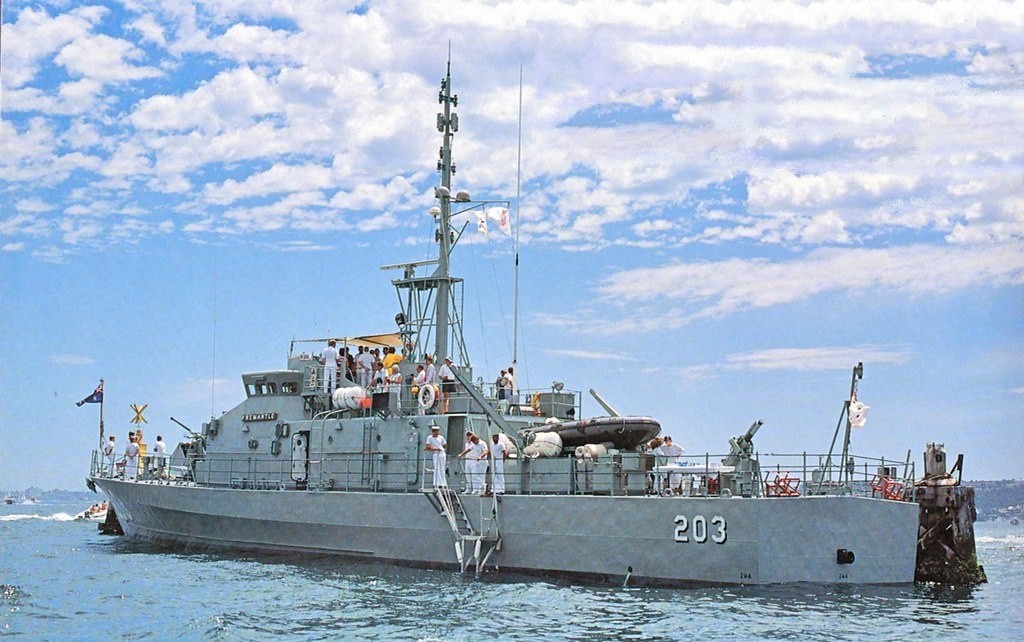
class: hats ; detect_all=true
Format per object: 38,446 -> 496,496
467,431 -> 473,434
444,356 -> 453,363
492,433 -> 499,435
431,426 -> 440,430
471,436 -> 479,440
664,436 -> 671,440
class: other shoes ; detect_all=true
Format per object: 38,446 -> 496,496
490,491 -> 503,494
478,491 -> 485,496
472,491 -> 478,495
462,490 -> 471,495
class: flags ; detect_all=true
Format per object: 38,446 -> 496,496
475,210 -> 489,233
486,207 -> 512,236
76,382 -> 103,407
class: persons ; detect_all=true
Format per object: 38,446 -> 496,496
125,432 -> 140,479
437,355 -> 456,413
388,364 -> 402,416
496,367 -> 514,401
89,500 -> 108,515
489,434 -> 508,495
321,341 -> 338,394
412,357 -> 437,414
367,362 -> 390,415
153,435 -> 167,474
648,436 -> 685,496
335,344 -> 409,388
103,435 -> 119,478
457,431 -> 490,495
425,426 -> 447,488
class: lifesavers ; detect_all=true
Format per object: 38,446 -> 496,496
344,386 -> 366,411
338,387 -> 352,409
417,384 -> 439,410
332,388 -> 343,409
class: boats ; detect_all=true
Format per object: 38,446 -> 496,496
77,35 -> 920,585
